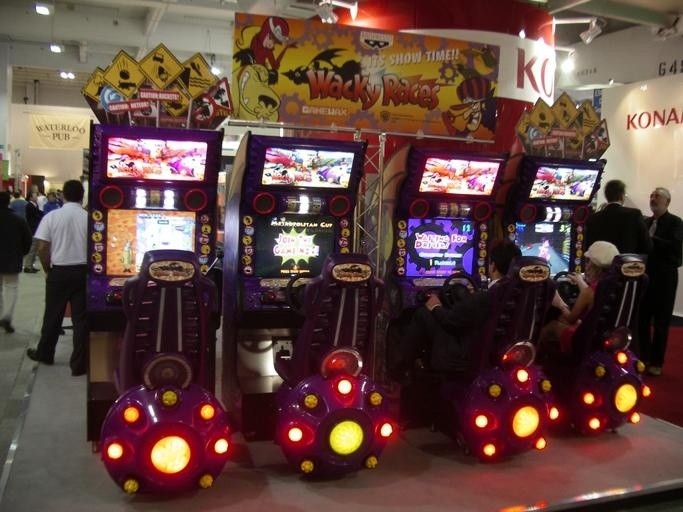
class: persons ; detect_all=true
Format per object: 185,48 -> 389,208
637,187 -> 683,375
586,180 -> 653,357
0,179 -> 88,377
386,237 -> 522,374
539,238 -> 621,357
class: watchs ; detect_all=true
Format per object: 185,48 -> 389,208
431,306 -> 439,311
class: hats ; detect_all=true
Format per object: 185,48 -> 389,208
584,240 -> 619,268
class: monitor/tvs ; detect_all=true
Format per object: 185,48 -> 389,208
405,218 -> 475,278
256,214 -> 336,277
107,208 -> 196,278
515,222 -> 572,277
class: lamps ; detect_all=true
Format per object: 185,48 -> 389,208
550,14 -> 606,45
312,0 -> 362,24
554,44 -> 578,75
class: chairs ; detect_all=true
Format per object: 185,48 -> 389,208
429,252 -> 553,397
111,242 -> 221,397
272,248 -> 391,392
557,249 -> 654,366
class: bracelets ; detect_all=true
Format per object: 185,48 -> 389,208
559,304 -> 568,310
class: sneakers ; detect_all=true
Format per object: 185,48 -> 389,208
27,348 -> 53,364
24,267 -> 39,273
0,320 -> 14,333
